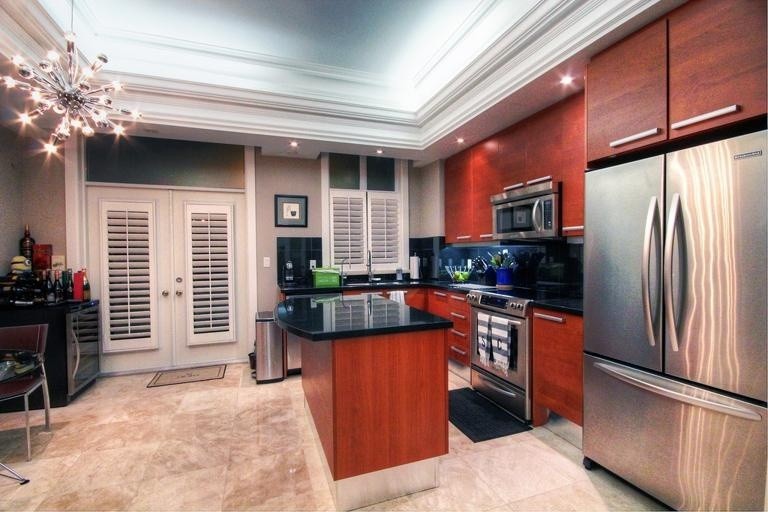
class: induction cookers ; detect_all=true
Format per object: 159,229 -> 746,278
465,288 -> 579,318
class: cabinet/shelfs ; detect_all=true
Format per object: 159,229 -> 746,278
0,300 -> 101,413
343,291 -> 384,299
496,104 -> 559,193
558,87 -> 586,237
427,289 -> 472,368
384,289 -> 426,311
443,136 -> 497,244
531,312 -> 584,426
586,1 -> 767,170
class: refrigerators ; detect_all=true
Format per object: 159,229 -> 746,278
580,124 -> 767,510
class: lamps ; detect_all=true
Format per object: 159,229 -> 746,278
0,0 -> 143,160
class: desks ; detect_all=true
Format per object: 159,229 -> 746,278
0,347 -> 49,487
270,292 -> 455,511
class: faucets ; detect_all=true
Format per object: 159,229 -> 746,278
366,250 -> 373,277
342,292 -> 349,310
366,294 -> 373,316
340,258 -> 351,283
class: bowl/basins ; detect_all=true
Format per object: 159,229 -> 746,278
444,265 -> 475,284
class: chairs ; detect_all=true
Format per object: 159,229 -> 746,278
0,323 -> 51,462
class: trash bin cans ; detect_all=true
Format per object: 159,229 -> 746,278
255,311 -> 284,384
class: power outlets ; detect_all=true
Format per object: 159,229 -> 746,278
310,260 -> 316,270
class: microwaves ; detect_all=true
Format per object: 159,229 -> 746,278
490,182 -> 560,240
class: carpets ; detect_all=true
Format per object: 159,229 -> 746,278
447,386 -> 535,444
147,362 -> 228,388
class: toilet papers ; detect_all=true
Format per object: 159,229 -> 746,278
409,257 -> 420,279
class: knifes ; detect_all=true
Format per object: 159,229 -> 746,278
471,255 -> 488,272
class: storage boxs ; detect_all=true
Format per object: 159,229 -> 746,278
313,266 -> 341,289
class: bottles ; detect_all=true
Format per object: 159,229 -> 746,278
38,267 -> 91,304
19,223 -> 36,260
396,268 -> 403,280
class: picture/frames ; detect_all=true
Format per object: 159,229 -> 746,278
274,195 -> 309,228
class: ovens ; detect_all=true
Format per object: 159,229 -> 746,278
469,307 -> 533,428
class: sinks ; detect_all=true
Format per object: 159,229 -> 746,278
346,282 -> 372,288
376,281 -> 404,288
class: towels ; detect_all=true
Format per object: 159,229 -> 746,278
490,315 -> 511,379
477,311 -> 490,369
390,292 -> 405,305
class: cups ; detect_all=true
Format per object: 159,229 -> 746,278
31,243 -> 52,272
494,268 -> 514,291
